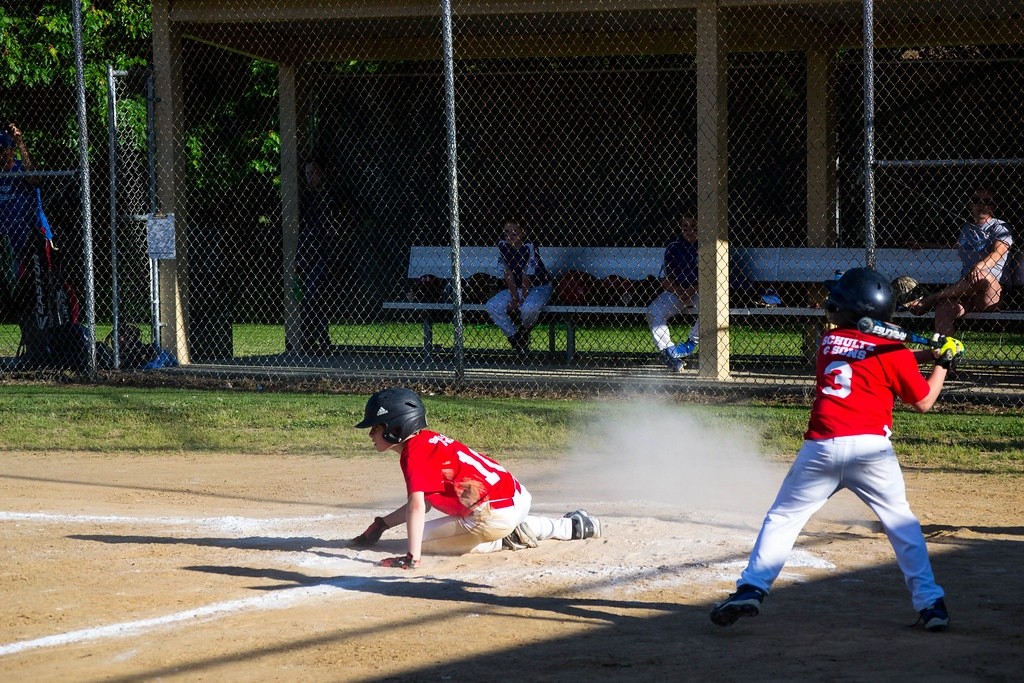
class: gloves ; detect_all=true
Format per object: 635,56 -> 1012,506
350,516 -> 388,549
930,332 -> 965,370
377,551 -> 416,570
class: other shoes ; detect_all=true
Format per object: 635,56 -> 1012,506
906,299 -> 932,316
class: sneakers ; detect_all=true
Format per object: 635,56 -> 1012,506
563,509 -> 602,541
666,343 -> 694,358
710,583 -> 765,628
669,355 -> 686,373
502,523 -> 541,550
920,598 -> 950,630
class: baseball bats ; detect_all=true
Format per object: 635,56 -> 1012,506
857,317 -> 942,348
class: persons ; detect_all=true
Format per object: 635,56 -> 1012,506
486,219 -> 552,358
902,188 -> 1012,382
0,123 -> 52,256
711,266 -> 949,629
349,386 -> 601,569
296,159 -> 338,355
646,214 -> 699,371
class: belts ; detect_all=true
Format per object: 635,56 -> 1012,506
489,480 -> 521,509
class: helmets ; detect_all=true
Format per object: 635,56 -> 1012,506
353,386 -> 430,444
824,268 -> 894,323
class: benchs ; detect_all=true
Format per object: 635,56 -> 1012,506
384,246 -> 1024,367
